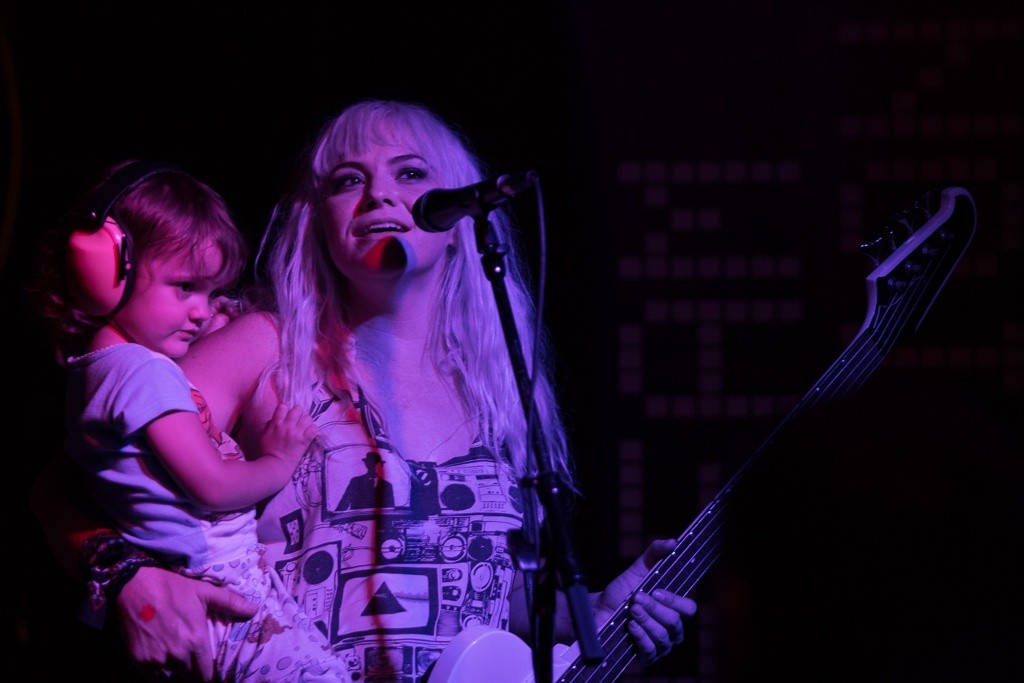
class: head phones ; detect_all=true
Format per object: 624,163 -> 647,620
55,161 -> 189,319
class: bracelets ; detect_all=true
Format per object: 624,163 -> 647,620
76,530 -> 162,613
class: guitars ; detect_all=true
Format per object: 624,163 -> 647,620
424,179 -> 980,683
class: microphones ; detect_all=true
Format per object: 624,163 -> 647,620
412,169 -> 537,234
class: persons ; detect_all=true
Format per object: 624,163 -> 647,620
85,95 -> 701,683
51,161 -> 358,683
